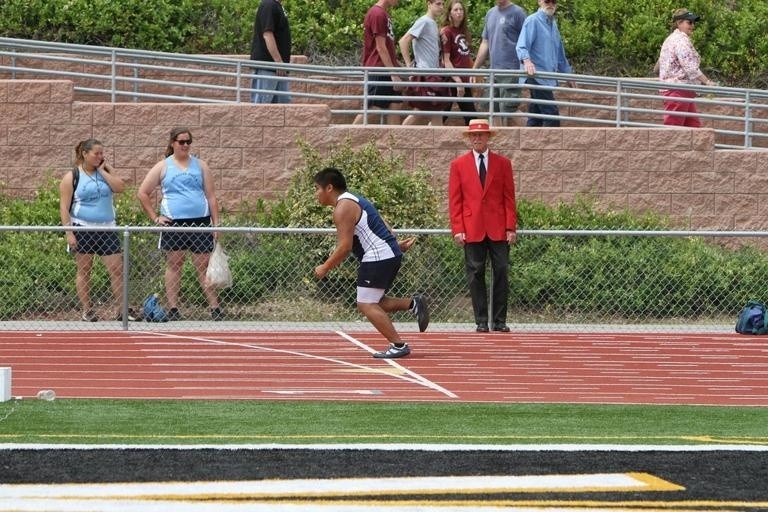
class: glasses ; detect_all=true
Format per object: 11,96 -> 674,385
175,139 -> 192,145
545,0 -> 558,4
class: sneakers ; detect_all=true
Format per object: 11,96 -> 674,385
114,308 -> 142,321
81,310 -> 104,321
210,308 -> 223,320
409,295 -> 429,332
168,308 -> 183,321
373,343 -> 410,358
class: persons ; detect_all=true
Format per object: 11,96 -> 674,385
515,0 -> 576,127
450,119 -> 516,332
399,0 -> 446,125
313,168 -> 429,358
137,128 -> 222,320
60,139 -> 135,322
653,10 -> 715,126
469,0 -> 527,126
351,0 -> 401,124
251,0 -> 291,103
441,0 -> 477,126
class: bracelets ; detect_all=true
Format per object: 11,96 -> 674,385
707,80 -> 710,85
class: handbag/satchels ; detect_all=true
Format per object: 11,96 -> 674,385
735,305 -> 768,335
408,76 -> 453,111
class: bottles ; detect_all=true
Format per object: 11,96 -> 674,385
36,390 -> 56,401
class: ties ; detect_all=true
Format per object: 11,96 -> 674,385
479,155 -> 486,185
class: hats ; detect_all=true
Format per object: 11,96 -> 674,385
462,119 -> 496,137
672,12 -> 700,22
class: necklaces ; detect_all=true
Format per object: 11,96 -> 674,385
81,163 -> 100,193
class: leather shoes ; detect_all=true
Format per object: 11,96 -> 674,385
492,322 -> 509,332
477,323 -> 489,332
